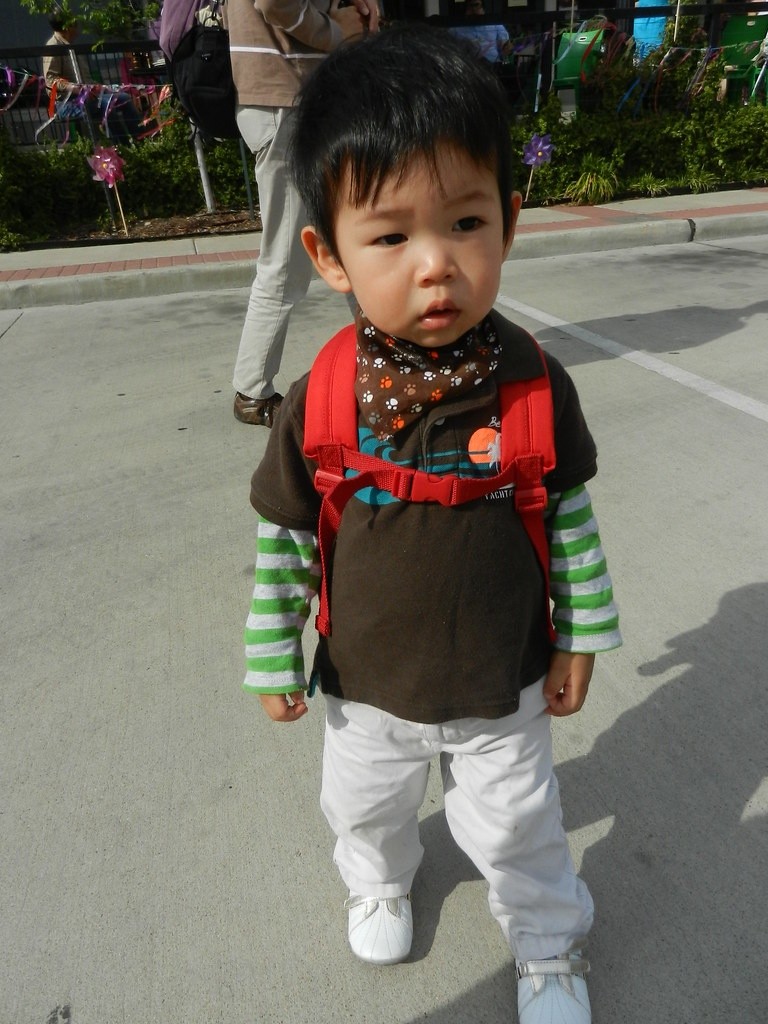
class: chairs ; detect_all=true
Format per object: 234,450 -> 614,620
718,14 -> 768,106
551,28 -> 605,121
44,86 -> 84,144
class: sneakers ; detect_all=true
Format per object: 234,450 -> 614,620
513,953 -> 592,1024
234,388 -> 283,428
347,888 -> 411,964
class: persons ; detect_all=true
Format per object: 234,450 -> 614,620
43,12 -> 93,102
225,0 -> 385,426
232,23 -> 625,1024
451,0 -> 508,69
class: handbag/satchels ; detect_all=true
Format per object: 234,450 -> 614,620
172,19 -> 241,138
